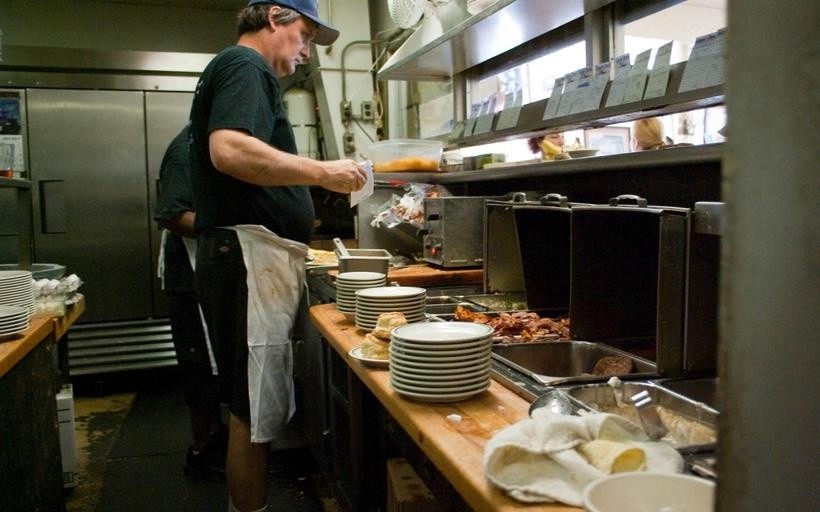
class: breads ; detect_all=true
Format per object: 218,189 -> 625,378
372,312 -> 408,340
360,333 -> 389,361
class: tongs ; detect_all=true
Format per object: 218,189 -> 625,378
631,388 -> 670,444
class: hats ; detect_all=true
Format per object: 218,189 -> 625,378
247,0 -> 340,47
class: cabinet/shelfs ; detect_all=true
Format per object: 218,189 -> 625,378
370,0 -> 729,188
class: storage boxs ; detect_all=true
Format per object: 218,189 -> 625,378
386,456 -> 444,512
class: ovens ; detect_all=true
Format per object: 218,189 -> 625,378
22,85 -> 195,378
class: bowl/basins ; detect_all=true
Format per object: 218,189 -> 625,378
582,469 -> 716,512
365,138 -> 445,174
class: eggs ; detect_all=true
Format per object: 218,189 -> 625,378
32,274 -> 78,291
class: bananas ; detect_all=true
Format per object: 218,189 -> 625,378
540,137 -> 581,160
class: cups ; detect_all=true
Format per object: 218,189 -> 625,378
585,441 -> 646,477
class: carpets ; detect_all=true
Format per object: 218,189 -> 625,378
97,458 -> 229,510
107,385 -> 231,456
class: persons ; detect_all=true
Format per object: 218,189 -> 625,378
156,122 -> 300,482
194,0 -> 369,511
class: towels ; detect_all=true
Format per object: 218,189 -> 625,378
477,404 -> 690,510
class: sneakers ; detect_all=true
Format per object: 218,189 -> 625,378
208,422 -> 229,452
181,444 -> 227,482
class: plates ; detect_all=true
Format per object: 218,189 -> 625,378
637,441 -> 685,476
0,268 -> 38,343
564,149 -> 601,160
333,270 -> 493,406
